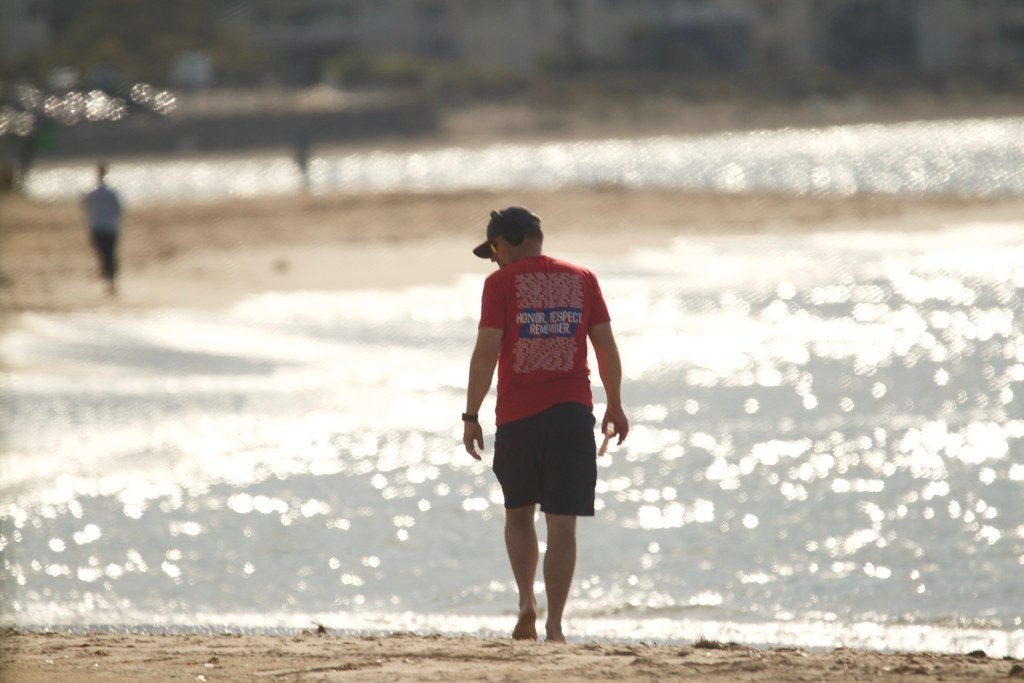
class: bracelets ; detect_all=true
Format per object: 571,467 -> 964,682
462,413 -> 479,420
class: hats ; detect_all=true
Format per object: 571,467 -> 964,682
474,206 -> 541,258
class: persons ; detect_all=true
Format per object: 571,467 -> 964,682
83,161 -> 121,296
464,207 -> 629,642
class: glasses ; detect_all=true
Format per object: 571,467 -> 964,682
489,235 -> 503,252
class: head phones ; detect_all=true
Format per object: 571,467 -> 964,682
489,209 -> 526,246
